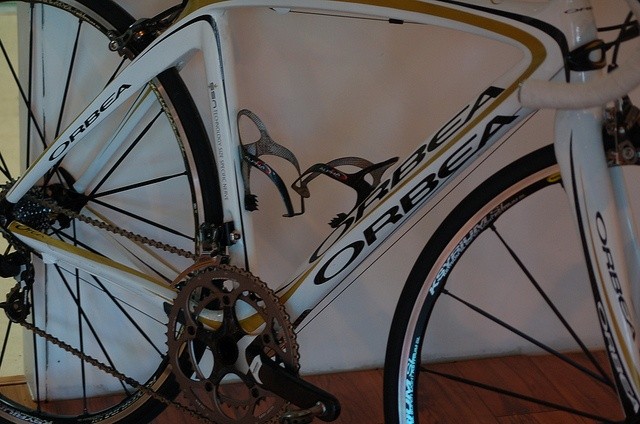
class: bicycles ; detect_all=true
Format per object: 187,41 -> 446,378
0,0 -> 640,423
